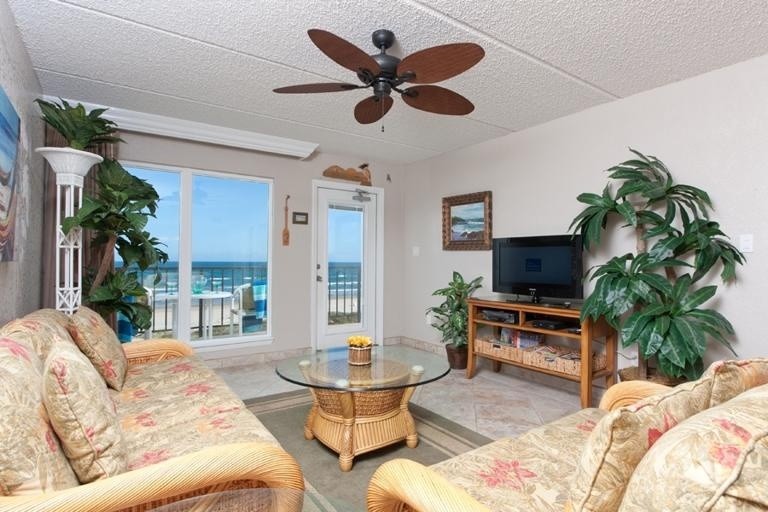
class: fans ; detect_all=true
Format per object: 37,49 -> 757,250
271,27 -> 486,133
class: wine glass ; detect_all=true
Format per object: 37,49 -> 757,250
212,281 -> 221,294
167,282 -> 177,295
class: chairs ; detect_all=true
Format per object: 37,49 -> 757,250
133,285 -> 153,345
229,282 -> 267,335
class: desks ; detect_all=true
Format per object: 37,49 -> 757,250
162,288 -> 234,339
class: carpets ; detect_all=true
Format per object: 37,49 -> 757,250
241,386 -> 498,512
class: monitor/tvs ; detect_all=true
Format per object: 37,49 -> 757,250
492,234 -> 584,307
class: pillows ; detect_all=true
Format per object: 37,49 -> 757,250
569,373 -> 712,510
701,358 -> 768,405
42,336 -> 129,487
67,302 -> 130,394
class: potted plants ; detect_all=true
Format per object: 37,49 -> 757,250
425,269 -> 482,370
567,145 -> 747,389
30,93 -> 127,187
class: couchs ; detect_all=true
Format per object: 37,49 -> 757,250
367,353 -> 767,512
0,299 -> 306,512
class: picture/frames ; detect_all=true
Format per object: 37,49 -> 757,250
441,190 -> 493,252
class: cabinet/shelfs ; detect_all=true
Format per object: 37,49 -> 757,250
464,296 -> 618,410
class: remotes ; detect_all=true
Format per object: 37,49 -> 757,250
563,300 -> 571,307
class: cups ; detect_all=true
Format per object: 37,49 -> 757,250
191,275 -> 207,294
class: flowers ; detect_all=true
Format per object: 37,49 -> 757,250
348,334 -> 377,351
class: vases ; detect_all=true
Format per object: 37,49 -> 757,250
346,346 -> 372,366
